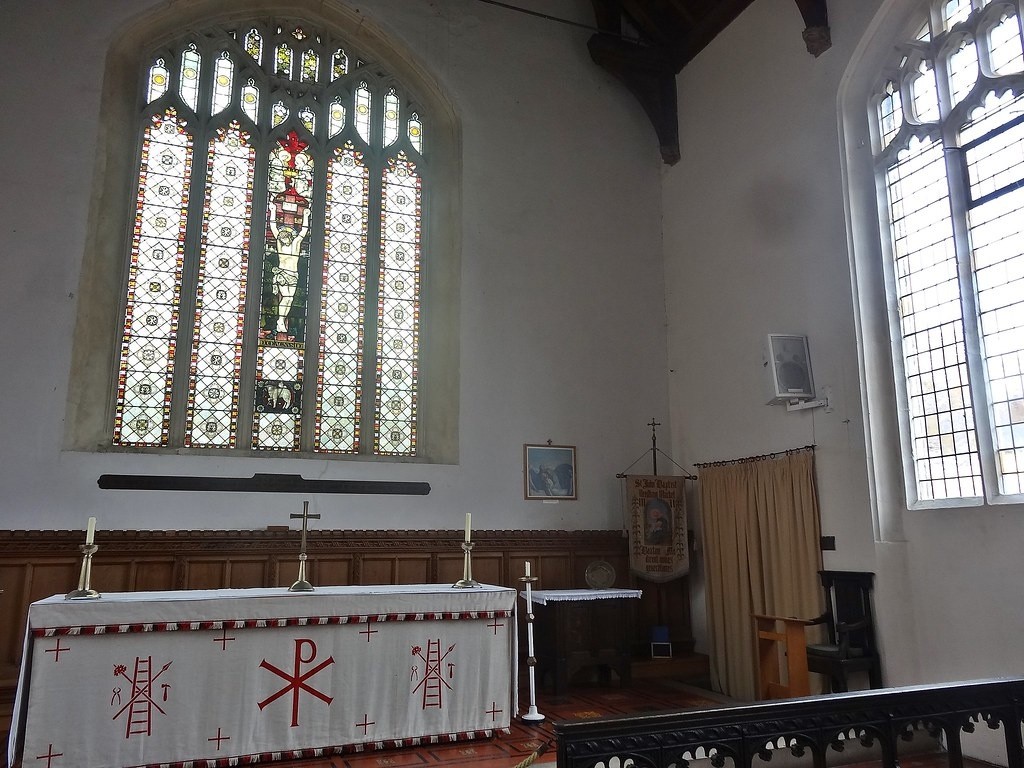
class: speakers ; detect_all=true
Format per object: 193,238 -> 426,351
761,334 -> 816,406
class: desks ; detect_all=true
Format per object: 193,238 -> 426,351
750,615 -> 814,702
5,580 -> 520,768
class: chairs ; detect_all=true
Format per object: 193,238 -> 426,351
803,568 -> 881,694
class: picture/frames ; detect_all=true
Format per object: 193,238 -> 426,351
523,443 -> 577,500
766,334 -> 816,398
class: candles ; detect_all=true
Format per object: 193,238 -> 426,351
525,561 -> 530,578
85,516 -> 96,545
465,513 -> 472,541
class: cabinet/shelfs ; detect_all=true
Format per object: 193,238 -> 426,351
518,590 -> 642,697
